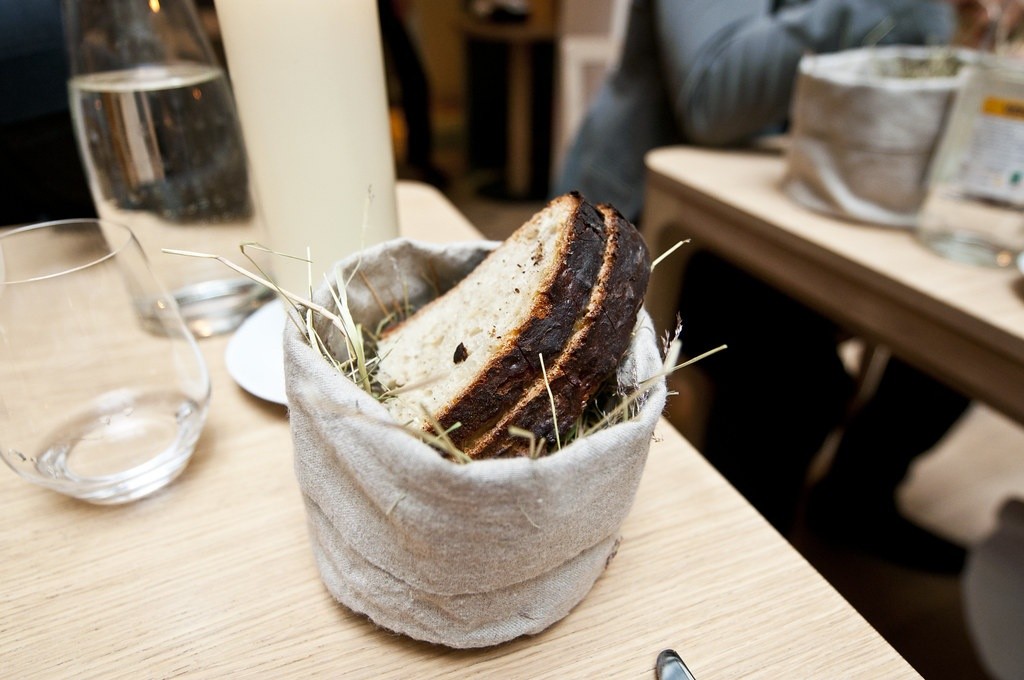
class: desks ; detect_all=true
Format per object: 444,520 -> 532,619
0,181 -> 923,680
635,135 -> 1023,452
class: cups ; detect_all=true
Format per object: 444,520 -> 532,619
0,219 -> 212,507
62,0 -> 275,341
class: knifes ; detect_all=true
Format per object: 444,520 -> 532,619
656,649 -> 695,680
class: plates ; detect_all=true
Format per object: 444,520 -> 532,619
224,298 -> 290,406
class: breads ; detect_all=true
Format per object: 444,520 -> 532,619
450,202 -> 650,460
379,190 -> 606,458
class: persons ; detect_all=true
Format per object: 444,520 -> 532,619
557,0 -> 1024,573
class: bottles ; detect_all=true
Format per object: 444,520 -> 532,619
911,0 -> 1024,271
